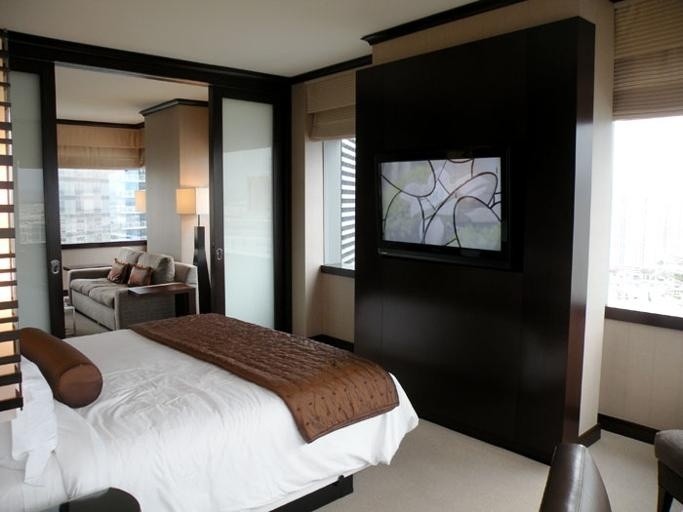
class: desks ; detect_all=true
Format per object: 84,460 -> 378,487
128,284 -> 196,317
63,264 -> 113,302
654,429 -> 682,512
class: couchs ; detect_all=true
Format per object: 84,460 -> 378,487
67,247 -> 200,330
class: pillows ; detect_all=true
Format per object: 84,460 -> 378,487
127,263 -> 152,288
107,256 -> 126,284
138,251 -> 176,282
20,324 -> 102,406
0,354 -> 57,489
45,412 -> 108,496
116,248 -> 139,268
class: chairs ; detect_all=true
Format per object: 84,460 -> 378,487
538,442 -> 611,511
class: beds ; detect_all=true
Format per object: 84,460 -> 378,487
0,311 -> 419,512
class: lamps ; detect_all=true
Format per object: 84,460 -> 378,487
176,186 -> 211,314
135,191 -> 146,213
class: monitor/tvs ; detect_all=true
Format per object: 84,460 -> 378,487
375,148 -> 511,269
